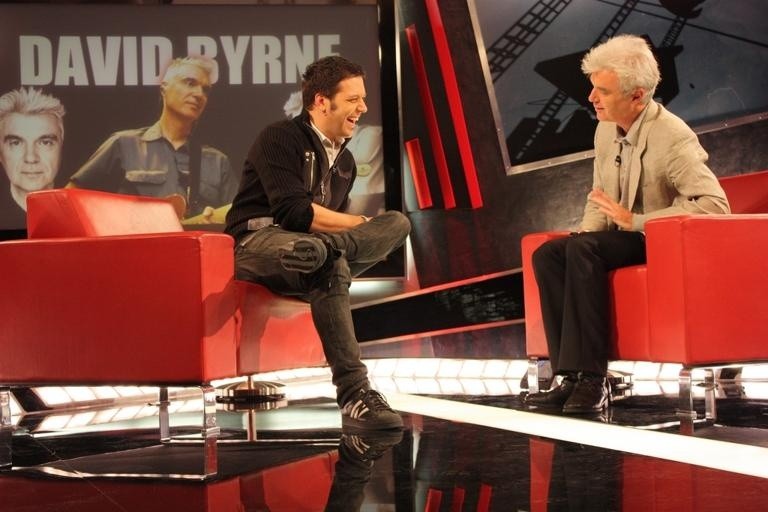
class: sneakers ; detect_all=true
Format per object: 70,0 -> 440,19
341,389 -> 403,430
340,433 -> 403,462
278,235 -> 329,273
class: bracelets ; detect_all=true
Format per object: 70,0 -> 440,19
361,214 -> 369,224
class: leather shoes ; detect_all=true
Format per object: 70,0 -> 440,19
563,374 -> 609,413
522,376 -> 573,408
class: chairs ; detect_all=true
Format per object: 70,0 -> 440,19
520,169 -> 768,435
1,188 -> 342,483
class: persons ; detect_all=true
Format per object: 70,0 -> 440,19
0,88 -> 70,242
64,54 -> 239,226
323,434 -> 409,512
523,33 -> 733,415
281,86 -> 384,214
222,54 -> 411,434
